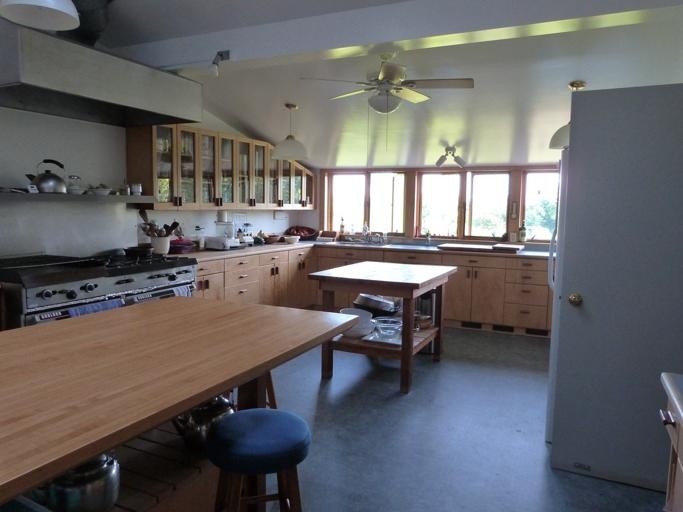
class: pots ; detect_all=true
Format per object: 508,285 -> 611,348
352,299 -> 398,316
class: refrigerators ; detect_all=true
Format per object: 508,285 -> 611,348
545,82 -> 683,492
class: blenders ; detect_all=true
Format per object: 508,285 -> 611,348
232,213 -> 254,247
206,211 -> 240,250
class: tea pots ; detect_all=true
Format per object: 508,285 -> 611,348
34,451 -> 121,511
170,388 -> 235,445
24,158 -> 67,194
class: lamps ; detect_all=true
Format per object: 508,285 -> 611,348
435,146 -> 465,168
0,0 -> 80,32
206,48 -> 231,79
368,46 -> 403,113
547,80 -> 585,150
270,101 -> 310,162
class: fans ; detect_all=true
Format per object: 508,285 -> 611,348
298,71 -> 476,105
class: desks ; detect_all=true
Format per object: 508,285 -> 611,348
307,261 -> 459,394
0,295 -> 359,511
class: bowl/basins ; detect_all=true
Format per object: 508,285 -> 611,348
284,236 -> 300,244
92,188 -> 112,196
69,187 -> 88,195
151,237 -> 170,254
340,308 -> 403,341
262,235 -> 280,245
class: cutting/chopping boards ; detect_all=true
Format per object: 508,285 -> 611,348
436,242 -> 520,254
492,243 -> 525,250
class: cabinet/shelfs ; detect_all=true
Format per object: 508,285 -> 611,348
657,373 -> 683,511
125,123 -> 316,211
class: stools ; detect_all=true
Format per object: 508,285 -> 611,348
203,408 -> 311,511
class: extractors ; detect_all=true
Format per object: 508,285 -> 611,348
0,16 -> 202,127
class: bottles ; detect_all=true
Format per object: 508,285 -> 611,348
519,220 -> 527,241
340,216 -> 344,234
363,222 -> 368,232
120,182 -> 143,196
425,229 -> 431,245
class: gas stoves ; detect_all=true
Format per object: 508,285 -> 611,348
2,246 -> 198,315
